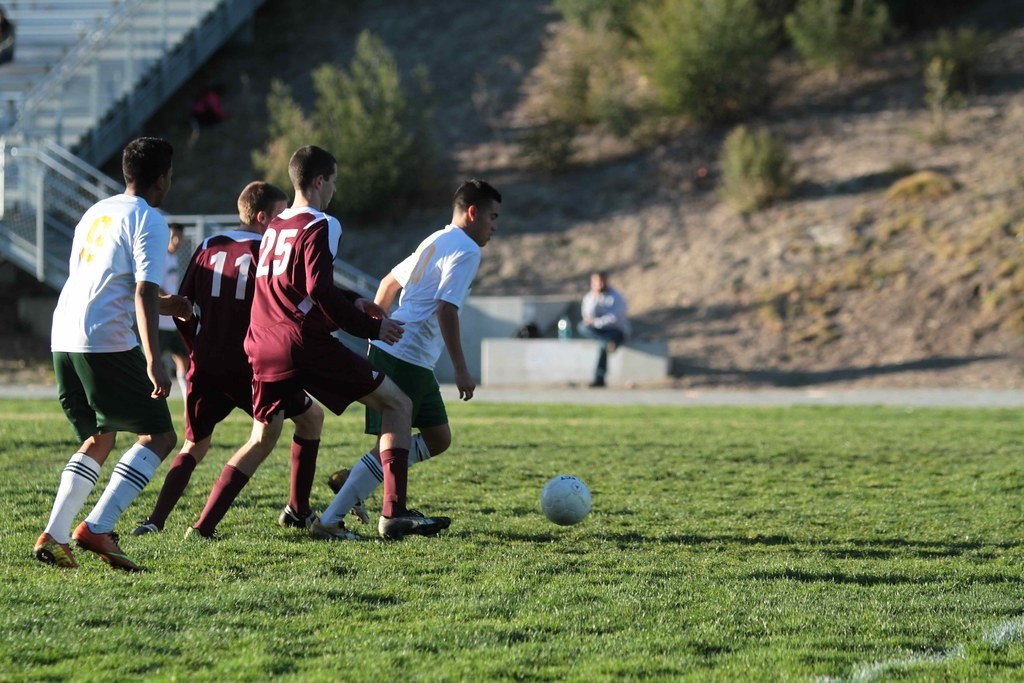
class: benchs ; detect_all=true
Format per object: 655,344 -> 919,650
480,338 -> 668,386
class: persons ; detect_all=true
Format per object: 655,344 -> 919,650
130,181 -> 326,536
34,137 -> 174,571
579,273 -> 630,388
139,223 -> 189,413
184,145 -> 452,543
310,179 -> 500,542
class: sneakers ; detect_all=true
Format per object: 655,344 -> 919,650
184,526 -> 221,541
378,509 -> 451,542
278,505 -> 321,527
134,523 -> 161,536
328,468 -> 369,524
308,516 -> 363,542
72,520 -> 138,571
32,531 -> 79,569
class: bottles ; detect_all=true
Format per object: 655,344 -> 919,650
558,314 -> 572,339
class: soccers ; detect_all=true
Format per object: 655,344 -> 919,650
540,474 -> 592,526
